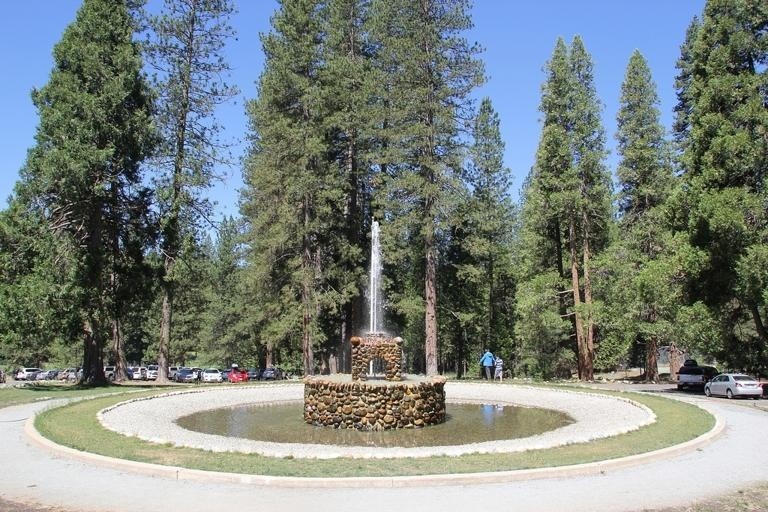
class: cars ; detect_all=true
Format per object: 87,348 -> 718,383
0,370 -> 8,383
14,364 -> 288,385
703,372 -> 764,401
684,360 -> 697,366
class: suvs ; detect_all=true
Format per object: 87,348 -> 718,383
675,366 -> 718,390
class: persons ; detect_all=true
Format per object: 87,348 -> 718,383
193,368 -> 201,386
480,349 -> 497,380
231,366 -> 246,374
493,355 -> 504,383
